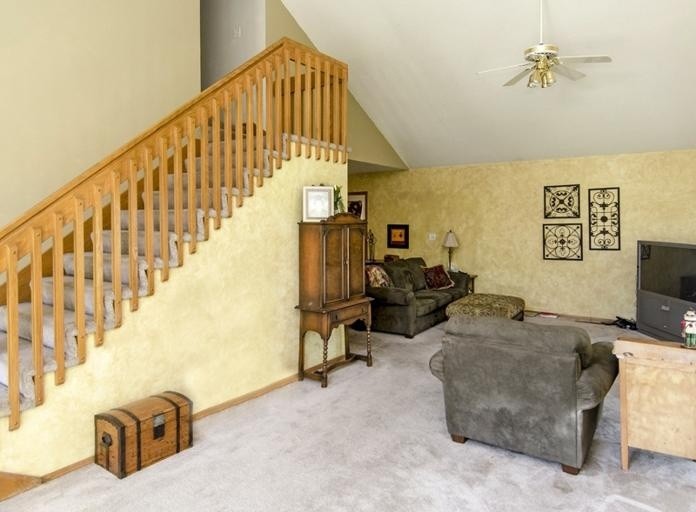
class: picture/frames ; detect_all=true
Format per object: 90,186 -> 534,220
348,191 -> 368,221
387,224 -> 410,249
303,186 -> 334,222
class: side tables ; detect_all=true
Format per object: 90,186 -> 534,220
467,274 -> 478,294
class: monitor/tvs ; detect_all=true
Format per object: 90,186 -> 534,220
635,239 -> 696,342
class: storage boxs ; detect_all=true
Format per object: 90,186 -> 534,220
94,390 -> 194,480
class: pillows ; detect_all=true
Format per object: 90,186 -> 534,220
419,264 -> 455,290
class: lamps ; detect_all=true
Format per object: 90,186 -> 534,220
442,229 -> 459,271
527,60 -> 556,89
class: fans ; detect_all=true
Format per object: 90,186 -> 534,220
477,1 -> 612,87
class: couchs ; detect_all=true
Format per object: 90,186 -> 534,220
353,256 -> 470,338
430,314 -> 620,476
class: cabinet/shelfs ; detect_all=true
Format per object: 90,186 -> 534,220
298,212 -> 375,387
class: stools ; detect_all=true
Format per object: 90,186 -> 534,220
444,293 -> 525,323
